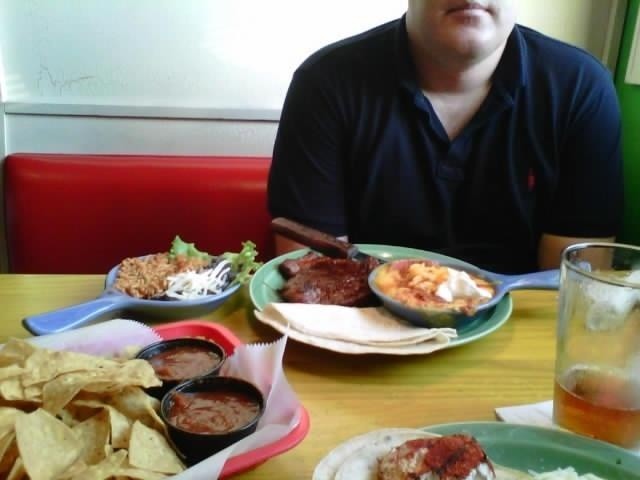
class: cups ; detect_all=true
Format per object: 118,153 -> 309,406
553,242 -> 640,451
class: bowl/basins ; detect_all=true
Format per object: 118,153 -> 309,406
135,338 -> 265,463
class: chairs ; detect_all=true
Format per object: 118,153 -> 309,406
0,152 -> 274,274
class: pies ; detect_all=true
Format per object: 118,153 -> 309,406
253,302 -> 458,355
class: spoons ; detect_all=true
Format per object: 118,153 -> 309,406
369,262 -> 590,322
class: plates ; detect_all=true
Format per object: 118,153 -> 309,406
18,252 -> 243,333
402,418 -> 640,479
250,242 -> 514,348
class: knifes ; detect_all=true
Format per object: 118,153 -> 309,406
269,217 -> 386,265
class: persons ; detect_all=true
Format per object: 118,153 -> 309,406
266,0 -> 622,271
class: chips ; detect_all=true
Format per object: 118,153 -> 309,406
0,337 -> 186,480
